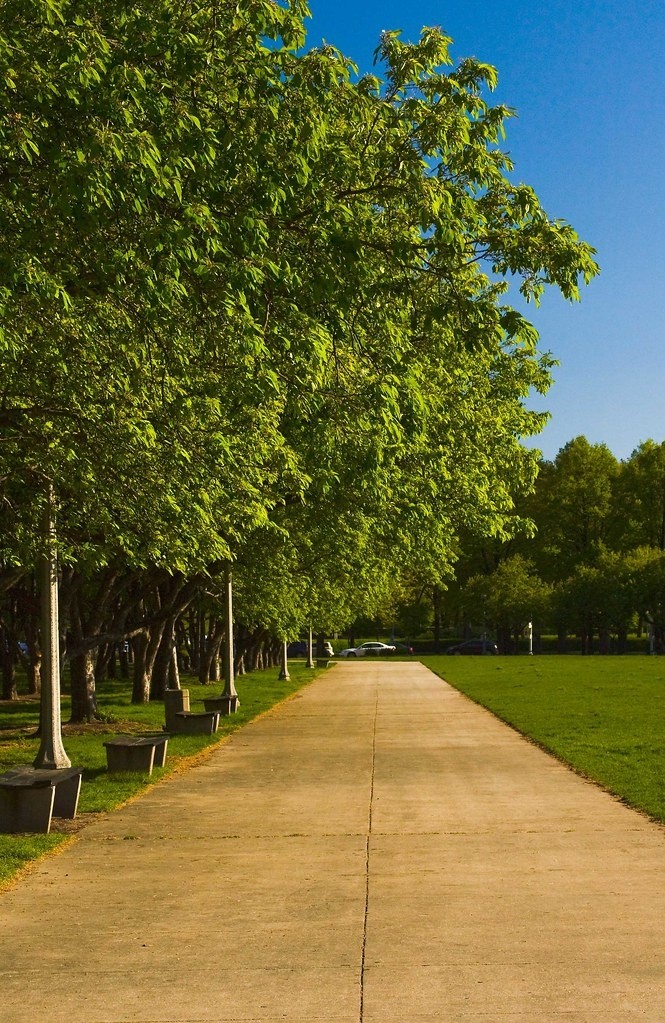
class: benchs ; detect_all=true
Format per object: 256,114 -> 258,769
178,710 -> 223,734
203,695 -> 238,715
0,768 -> 84,833
103,736 -> 169,774
316,658 -> 330,667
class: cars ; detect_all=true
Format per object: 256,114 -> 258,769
339,641 -> 396,658
440,639 -> 499,657
383,641 -> 414,656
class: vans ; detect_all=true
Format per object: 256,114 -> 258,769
287,640 -> 334,658
